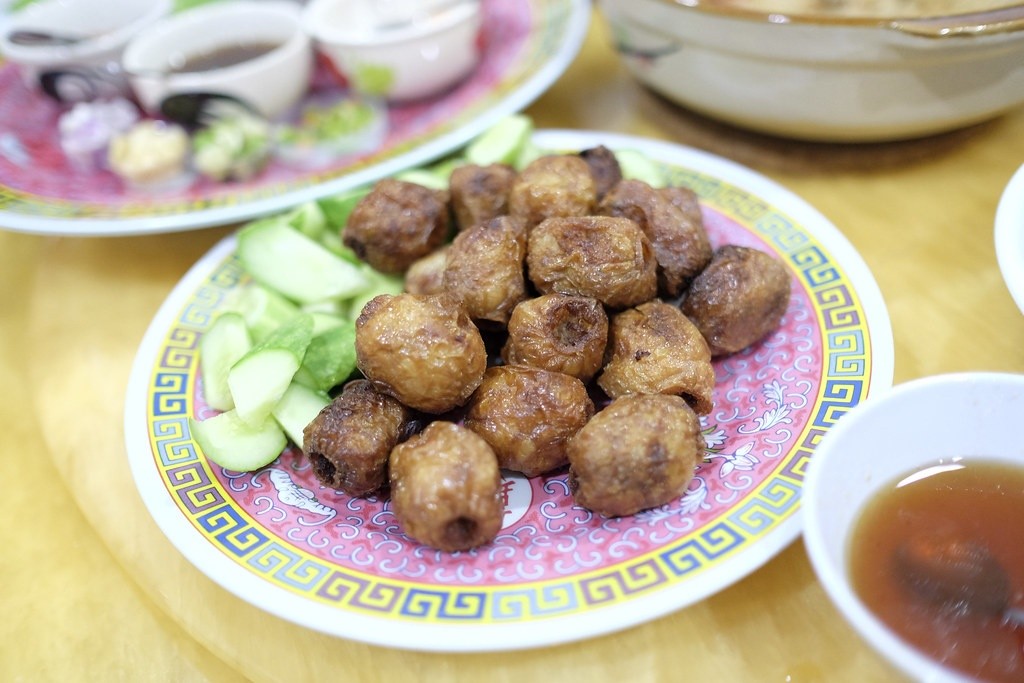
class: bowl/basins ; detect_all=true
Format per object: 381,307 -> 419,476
120,0 -> 316,120
800,370 -> 1024,683
303,0 -> 485,104
0,0 -> 175,105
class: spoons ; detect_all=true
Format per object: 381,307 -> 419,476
160,93 -> 257,127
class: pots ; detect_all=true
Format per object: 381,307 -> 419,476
593,0 -> 1024,145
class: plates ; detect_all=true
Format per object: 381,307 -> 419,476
122,127 -> 895,652
0,0 -> 589,236
